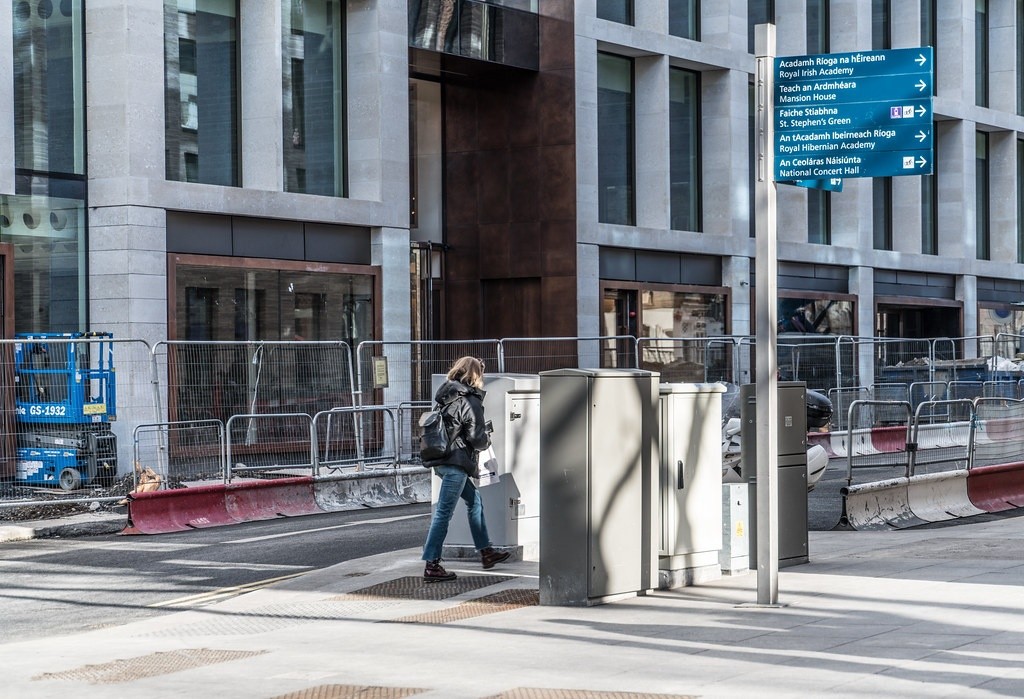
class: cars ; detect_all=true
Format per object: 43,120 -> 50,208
777,331 -> 853,367
657,362 -> 707,383
777,362 -> 858,390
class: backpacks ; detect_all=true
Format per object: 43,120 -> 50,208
418,396 -> 471,468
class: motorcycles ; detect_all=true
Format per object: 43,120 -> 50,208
716,380 -> 834,493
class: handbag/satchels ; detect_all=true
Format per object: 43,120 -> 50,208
471,446 -> 500,488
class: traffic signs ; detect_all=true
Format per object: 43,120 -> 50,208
772,45 -> 934,192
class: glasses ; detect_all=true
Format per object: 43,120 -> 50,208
478,357 -> 486,368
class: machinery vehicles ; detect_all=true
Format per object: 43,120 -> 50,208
15,330 -> 118,489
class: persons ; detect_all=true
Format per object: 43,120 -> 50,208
421,357 -> 511,582
222,362 -> 282,436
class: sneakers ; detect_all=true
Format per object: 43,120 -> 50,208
481,551 -> 511,568
423,564 -> 457,580
